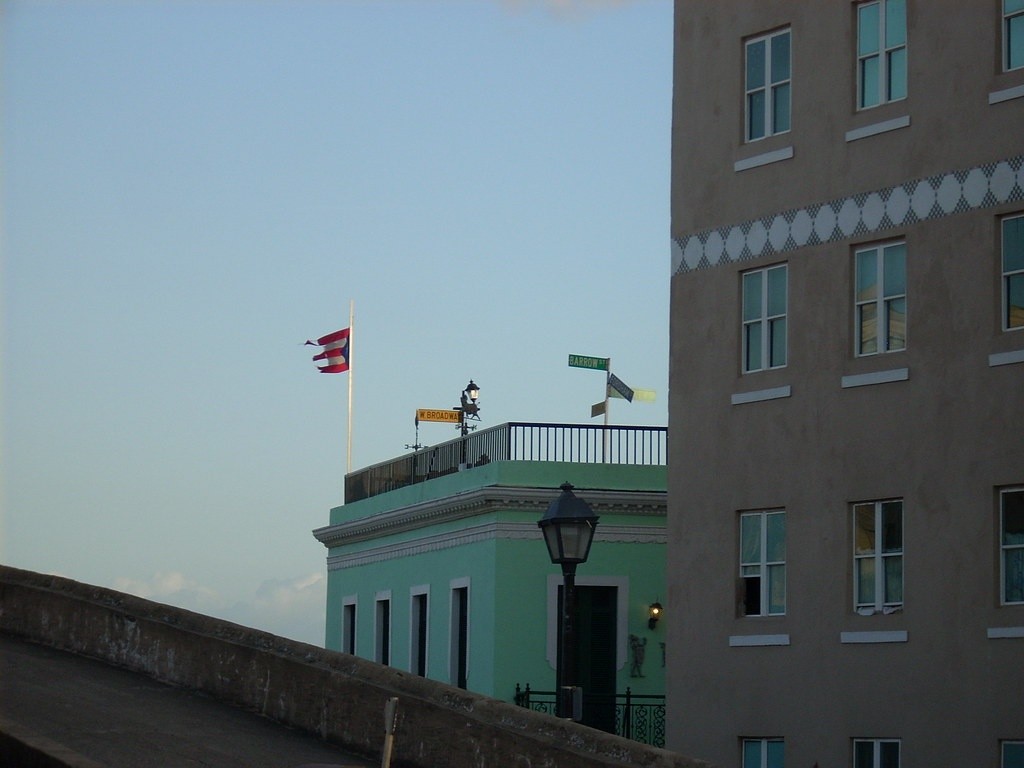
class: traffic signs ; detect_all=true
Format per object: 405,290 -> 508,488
567,354 -> 608,372
608,374 -> 633,403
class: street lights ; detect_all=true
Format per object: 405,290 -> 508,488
458,381 -> 480,471
535,483 -> 601,718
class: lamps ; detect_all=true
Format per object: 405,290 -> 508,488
647,602 -> 663,630
